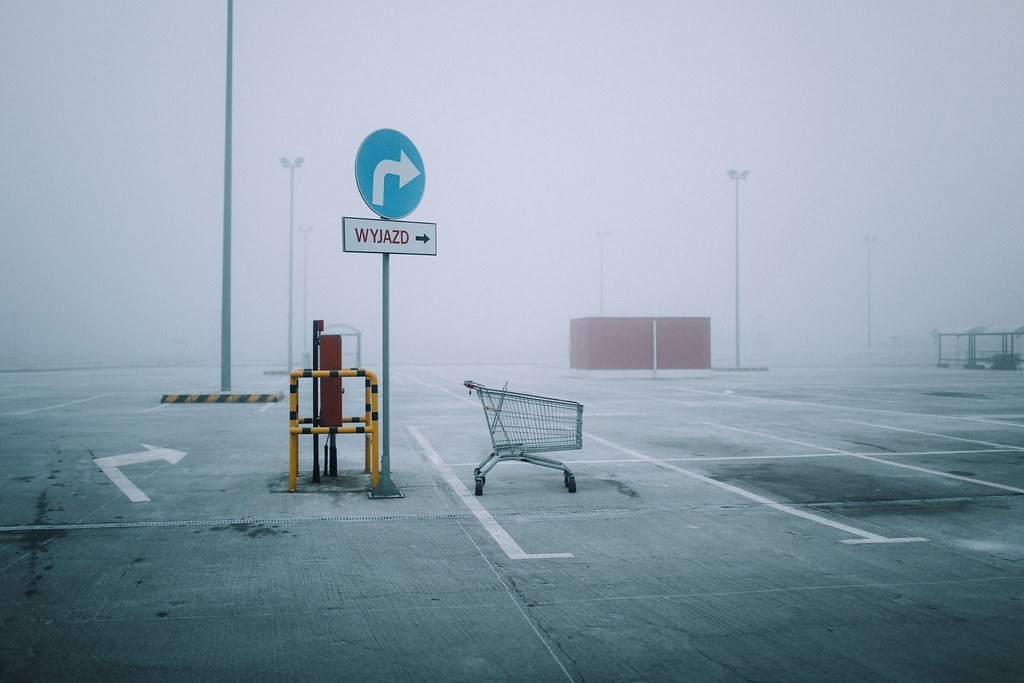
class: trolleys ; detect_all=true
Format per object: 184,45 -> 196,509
464,377 -> 584,495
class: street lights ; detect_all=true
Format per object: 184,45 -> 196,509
726,167 -> 752,367
278,153 -> 305,372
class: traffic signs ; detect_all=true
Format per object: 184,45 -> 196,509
341,216 -> 437,256
